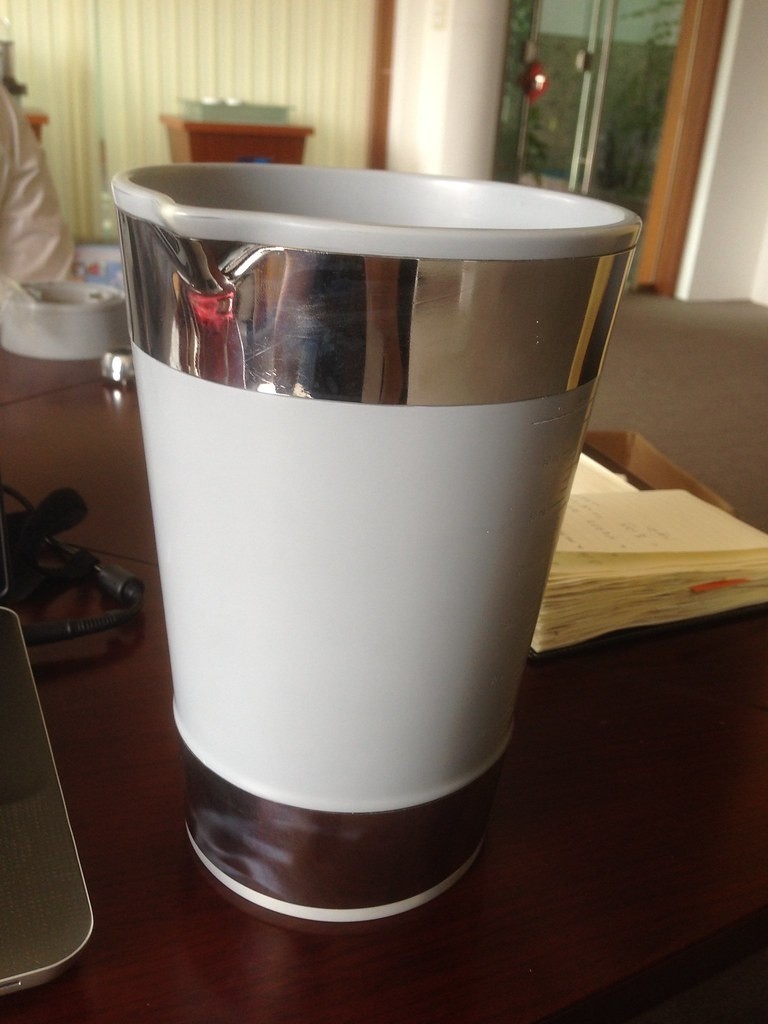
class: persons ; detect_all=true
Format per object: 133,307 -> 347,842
0,85 -> 75,282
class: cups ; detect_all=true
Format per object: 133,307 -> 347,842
109,161 -> 646,939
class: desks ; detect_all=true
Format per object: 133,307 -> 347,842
0,299 -> 768,1024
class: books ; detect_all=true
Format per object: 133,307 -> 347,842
531,452 -> 768,653
